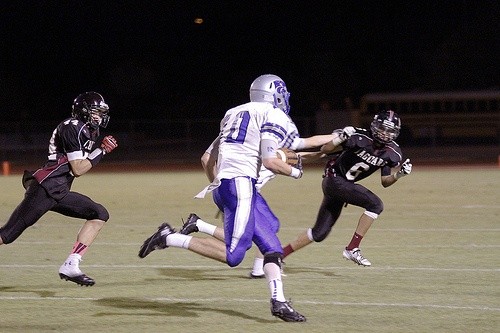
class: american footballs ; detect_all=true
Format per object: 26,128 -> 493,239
275,147 -> 299,168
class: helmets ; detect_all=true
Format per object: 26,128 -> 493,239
71,92 -> 108,129
249,74 -> 290,113
370,110 -> 401,145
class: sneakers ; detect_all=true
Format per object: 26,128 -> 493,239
280,259 -> 287,277
138,222 -> 175,258
343,247 -> 371,266
250,268 -> 265,277
59,261 -> 95,287
270,298 -> 306,322
179,213 -> 201,234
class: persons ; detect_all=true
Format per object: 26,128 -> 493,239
0,92 -> 118,286
280,110 -> 412,276
139,75 -> 342,322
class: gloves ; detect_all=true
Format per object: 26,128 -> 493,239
397,158 -> 411,176
290,165 -> 302,178
101,136 -> 118,154
339,126 -> 357,141
